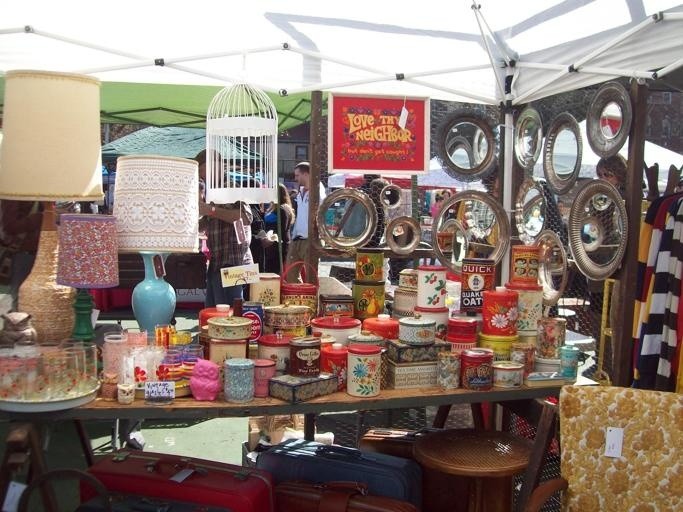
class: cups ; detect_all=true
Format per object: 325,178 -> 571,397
0,334 -> 97,398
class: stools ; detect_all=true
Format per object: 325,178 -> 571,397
414,425 -> 534,512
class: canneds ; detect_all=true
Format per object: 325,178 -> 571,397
493,361 -> 525,388
242,304 -> 262,343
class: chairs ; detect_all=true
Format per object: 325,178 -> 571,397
527,385 -> 683,512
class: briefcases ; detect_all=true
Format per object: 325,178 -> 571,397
86,431 -> 427,512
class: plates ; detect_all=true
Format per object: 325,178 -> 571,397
0,372 -> 101,413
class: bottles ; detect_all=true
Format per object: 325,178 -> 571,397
100,320 -> 207,385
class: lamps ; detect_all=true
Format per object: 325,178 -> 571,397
2,69 -> 105,346
56,213 -> 119,345
113,155 -> 201,340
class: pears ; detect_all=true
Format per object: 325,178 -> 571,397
367,294 -> 380,315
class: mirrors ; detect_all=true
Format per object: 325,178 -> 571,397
434,107 -> 498,183
535,230 -> 567,306
379,184 -> 403,209
432,189 -> 511,276
543,112 -> 582,196
586,81 -> 632,159
515,176 -> 547,247
386,216 -> 422,255
314,187 -> 378,251
567,179 -> 628,281
514,107 -> 543,169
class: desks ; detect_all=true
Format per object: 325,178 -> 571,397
0,378 -> 609,512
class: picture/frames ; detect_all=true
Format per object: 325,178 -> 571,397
328,93 -> 430,176
